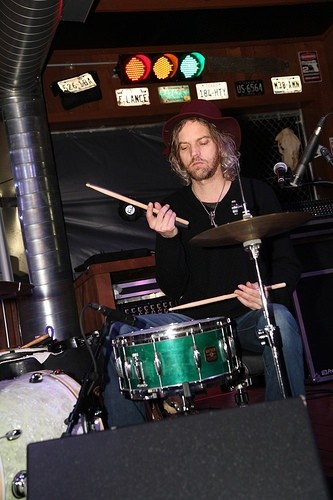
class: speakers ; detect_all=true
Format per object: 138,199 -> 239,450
28,395 -> 331,500
61,0 -> 94,23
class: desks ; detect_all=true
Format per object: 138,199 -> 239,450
73,253 -> 167,342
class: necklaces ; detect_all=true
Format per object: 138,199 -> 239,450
193,181 -> 225,226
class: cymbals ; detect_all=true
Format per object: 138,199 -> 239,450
191,211 -> 312,243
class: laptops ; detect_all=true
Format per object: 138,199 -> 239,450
74,248 -> 152,272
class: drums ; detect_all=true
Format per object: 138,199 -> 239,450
0,370 -> 106,499
112,317 -> 242,401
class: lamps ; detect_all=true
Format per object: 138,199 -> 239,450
118,51 -> 206,85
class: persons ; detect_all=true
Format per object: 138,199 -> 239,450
103,99 -> 305,427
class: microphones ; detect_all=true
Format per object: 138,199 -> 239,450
118,200 -> 190,229
289,116 -> 327,187
273,161 -> 289,183
89,303 -> 150,330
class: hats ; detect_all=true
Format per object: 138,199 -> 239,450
162,99 -> 242,157
